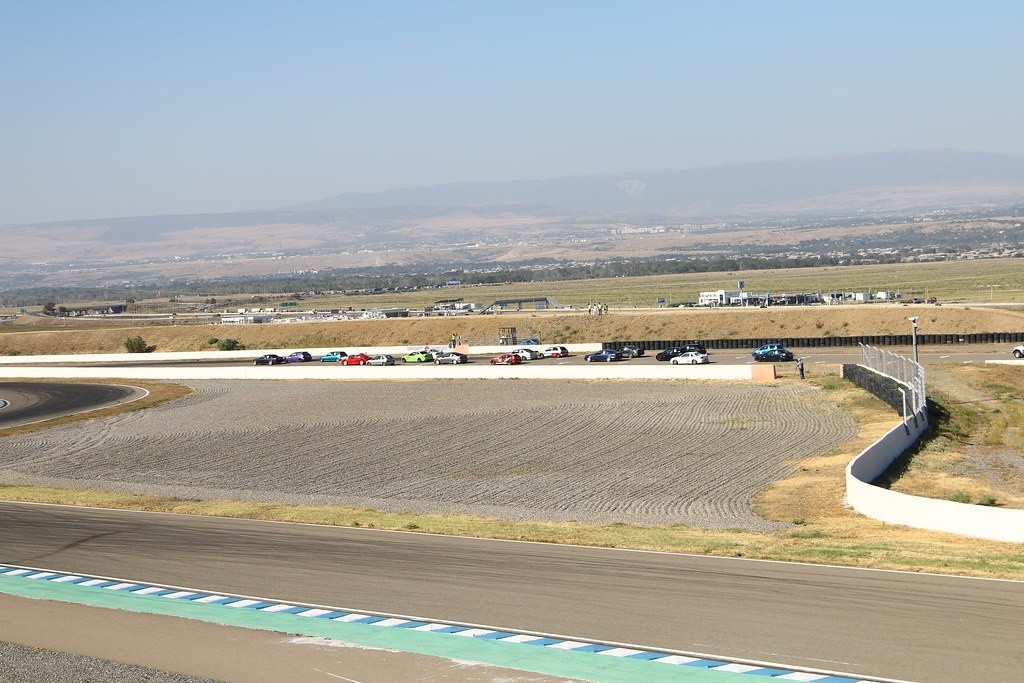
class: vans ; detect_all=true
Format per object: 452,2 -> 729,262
751,344 -> 783,356
655,347 -> 687,361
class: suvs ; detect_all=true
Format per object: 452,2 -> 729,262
543,346 -> 568,358
682,344 -> 707,354
366,354 -> 395,366
489,353 -> 521,365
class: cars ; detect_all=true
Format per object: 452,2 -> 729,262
754,349 -> 793,362
517,338 -> 542,345
913,298 -> 925,304
427,349 -> 443,362
252,355 -> 283,366
285,352 -> 312,363
669,352 -> 708,365
319,351 -> 348,363
584,349 -> 622,363
1012,345 -> 1024,358
434,352 -> 468,364
616,345 -> 645,358
341,353 -> 372,365
511,349 -> 536,361
401,351 -> 432,363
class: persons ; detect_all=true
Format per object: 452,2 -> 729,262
449,332 -> 458,348
588,302 -> 591,314
597,301 -> 602,317
593,303 -> 597,316
602,303 -> 608,315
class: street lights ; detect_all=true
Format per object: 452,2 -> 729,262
538,331 -> 541,345
